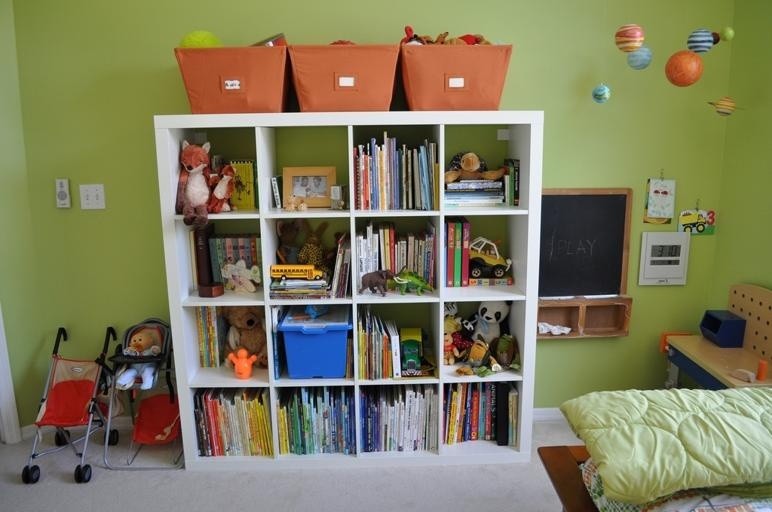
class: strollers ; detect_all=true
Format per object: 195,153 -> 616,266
19,327 -> 119,485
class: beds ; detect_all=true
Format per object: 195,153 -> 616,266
537,387 -> 772,511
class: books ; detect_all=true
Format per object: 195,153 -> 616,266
357,305 -> 402,379
277,386 -> 356,454
353,132 -> 439,210
444,383 -> 518,446
196,307 -> 232,366
193,389 -> 274,457
444,158 -> 520,207
444,216 -> 513,287
355,220 -> 437,289
270,232 -> 352,299
360,384 -> 439,452
190,227 -> 263,289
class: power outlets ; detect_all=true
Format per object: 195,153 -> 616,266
79,183 -> 106,211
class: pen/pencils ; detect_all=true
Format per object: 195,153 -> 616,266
288,316 -> 310,320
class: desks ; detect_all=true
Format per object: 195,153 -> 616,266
665,282 -> 772,389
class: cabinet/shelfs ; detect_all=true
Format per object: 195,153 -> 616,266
538,297 -> 634,339
151,109 -> 545,474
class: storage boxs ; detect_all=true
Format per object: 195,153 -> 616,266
277,306 -> 352,379
290,43 -> 399,112
173,45 -> 289,113
400,43 -> 514,112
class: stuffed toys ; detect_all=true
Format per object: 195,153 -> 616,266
173,140 -> 213,228
445,153 -> 506,183
223,306 -> 268,366
207,165 -> 236,213
277,219 -> 336,274
444,301 -> 520,376
399,26 -> 490,47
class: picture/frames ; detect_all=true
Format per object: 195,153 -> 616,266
281,164 -> 337,208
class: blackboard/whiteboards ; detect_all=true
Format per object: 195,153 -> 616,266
538,188 -> 633,300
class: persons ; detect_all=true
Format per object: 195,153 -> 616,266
293,176 -> 325,196
116,332 -> 162,392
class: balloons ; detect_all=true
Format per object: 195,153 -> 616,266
178,29 -> 225,49
